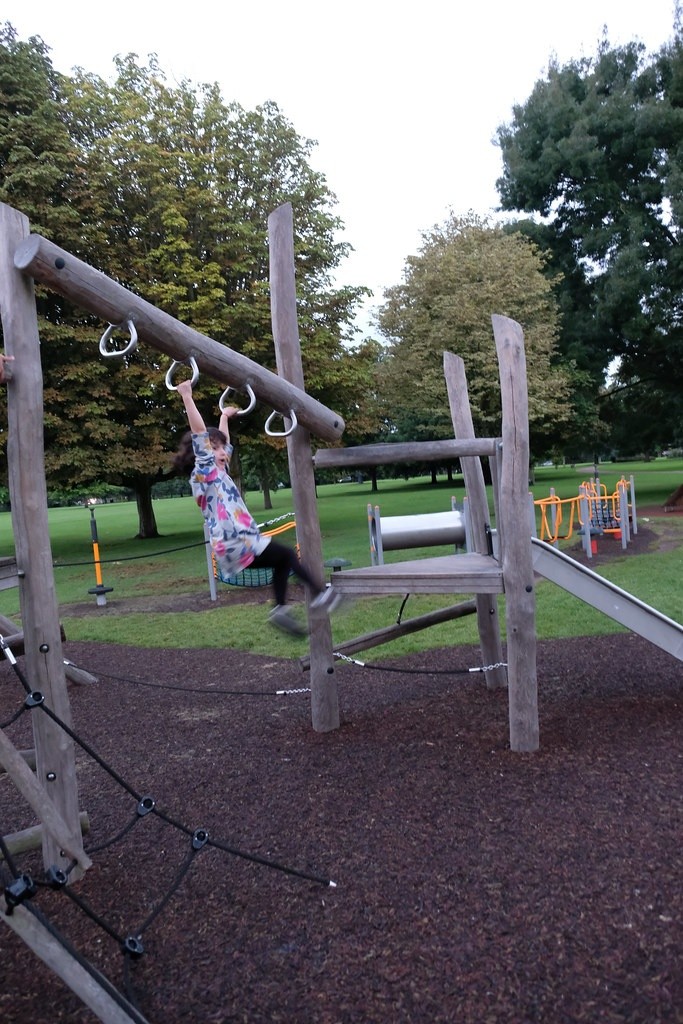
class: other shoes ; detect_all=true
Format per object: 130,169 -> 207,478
309,585 -> 343,615
268,604 -> 302,636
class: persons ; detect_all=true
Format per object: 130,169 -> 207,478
177,380 -> 347,641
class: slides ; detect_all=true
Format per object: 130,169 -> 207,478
531,533 -> 682,756
